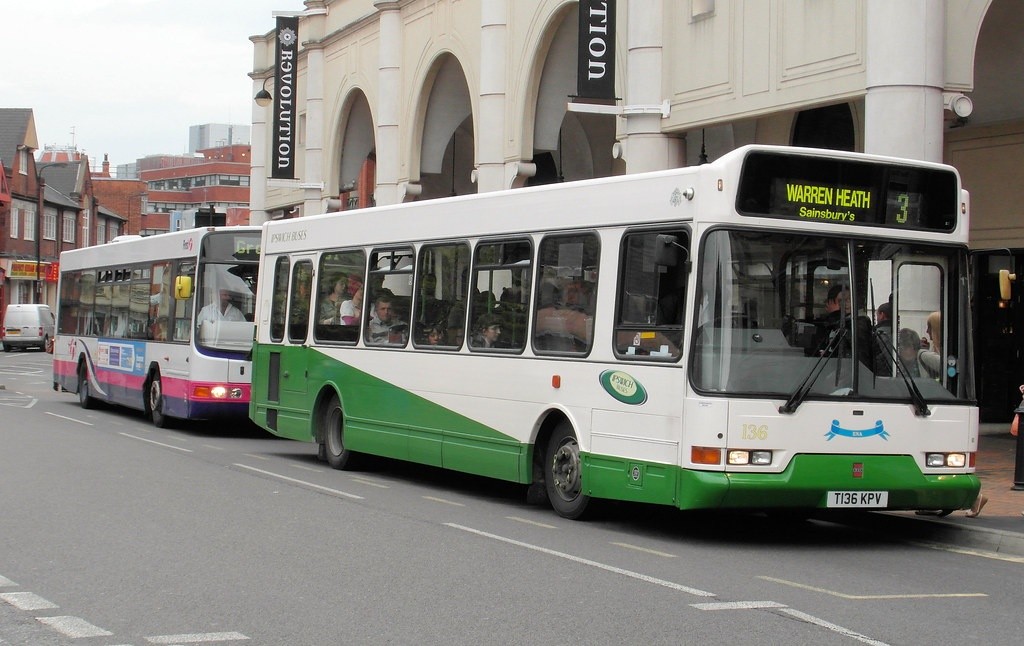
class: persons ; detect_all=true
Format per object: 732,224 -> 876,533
823,285 -> 899,374
340,275 -> 363,325
370,298 -> 408,344
322,272 -> 347,326
899,311 -> 988,520
282,271 -> 311,327
198,285 -> 248,321
539,258 -> 580,351
471,314 -> 503,348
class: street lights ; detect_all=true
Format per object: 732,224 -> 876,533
128,192 -> 150,234
34,162 -> 68,306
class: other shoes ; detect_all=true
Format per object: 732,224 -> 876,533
965,494 -> 988,516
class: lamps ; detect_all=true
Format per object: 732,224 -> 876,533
253,75 -> 277,108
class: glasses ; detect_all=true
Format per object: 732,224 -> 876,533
837,298 -> 851,303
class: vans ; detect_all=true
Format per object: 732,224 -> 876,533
3,305 -> 56,351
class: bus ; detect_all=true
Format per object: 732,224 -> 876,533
251,143 -> 1016,523
53,227 -> 262,427
